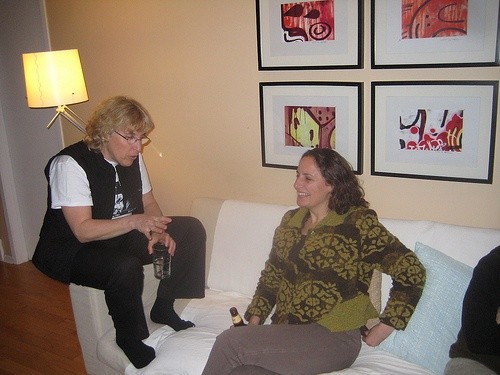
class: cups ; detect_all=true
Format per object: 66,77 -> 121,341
152,245 -> 172,279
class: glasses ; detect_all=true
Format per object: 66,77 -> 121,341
114,130 -> 150,145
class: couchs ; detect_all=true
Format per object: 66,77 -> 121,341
69,199 -> 500,375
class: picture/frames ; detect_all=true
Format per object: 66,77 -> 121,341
259,82 -> 364,175
256,0 -> 364,70
371,0 -> 500,69
371,81 -> 499,184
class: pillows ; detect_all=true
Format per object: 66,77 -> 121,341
377,243 -> 474,373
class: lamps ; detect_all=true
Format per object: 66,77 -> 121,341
20,47 -> 89,132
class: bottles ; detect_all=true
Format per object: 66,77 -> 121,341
229,307 -> 247,326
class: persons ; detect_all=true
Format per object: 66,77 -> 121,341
32,95 -> 207,369
203,149 -> 427,375
443,245 -> 500,375
